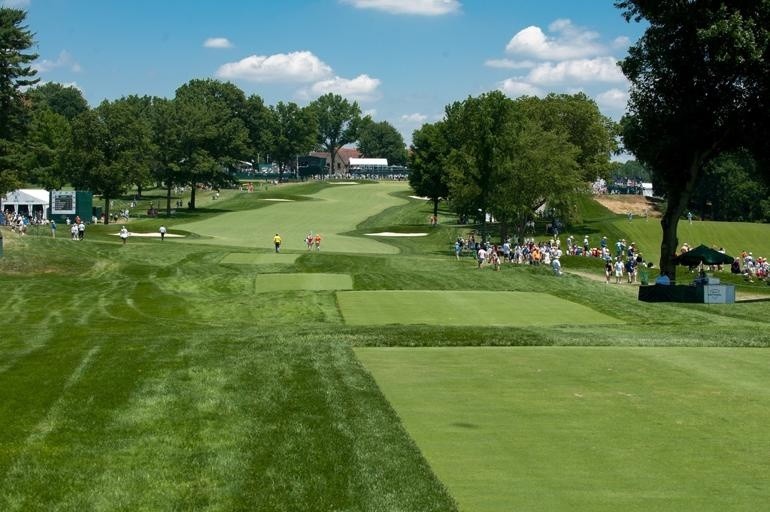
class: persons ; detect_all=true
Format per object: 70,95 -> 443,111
312,166 -> 408,183
274,233 -> 282,253
686,212 -> 692,224
314,234 -> 321,251
0,201 -> 167,246
172,174 -> 293,209
306,233 -> 314,249
456,213 -> 654,290
674,243 -> 770,286
